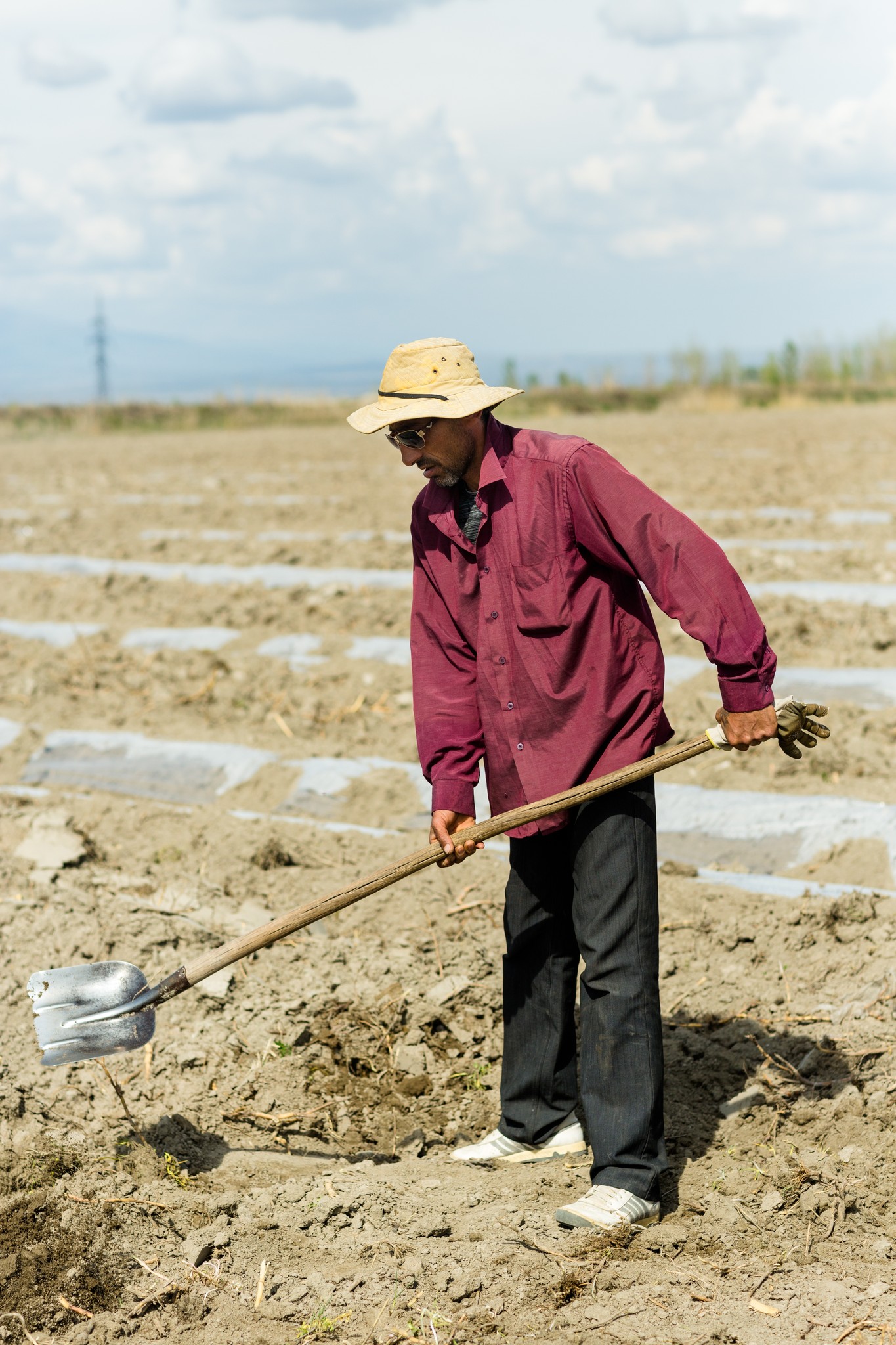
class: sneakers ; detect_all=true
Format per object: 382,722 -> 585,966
555,1184 -> 660,1229
449,1122 -> 587,1164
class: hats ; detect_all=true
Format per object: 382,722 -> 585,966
346,337 -> 525,434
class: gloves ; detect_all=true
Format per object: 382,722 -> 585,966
705,694 -> 831,759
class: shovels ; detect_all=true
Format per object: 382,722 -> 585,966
27,703 -> 807,1065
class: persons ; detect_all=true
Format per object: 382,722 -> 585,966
348,341 -> 779,1229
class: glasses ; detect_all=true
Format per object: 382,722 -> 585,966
385,417 -> 440,450
